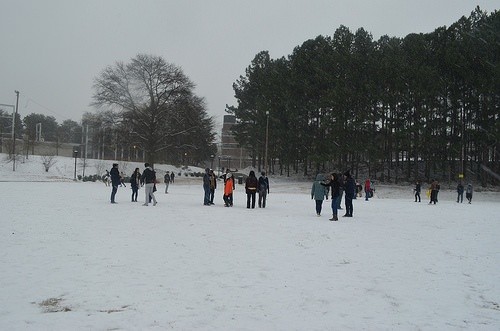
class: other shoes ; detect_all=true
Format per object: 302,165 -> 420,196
329,217 -> 338,221
141,203 -> 148,206
224,204 -> 230,207
343,214 -> 353,217
152,201 -> 158,206
111,202 -> 117,203
204,203 -> 210,206
135,200 -> 138,202
210,202 -> 215,205
131,201 -> 135,202
230,203 -> 234,207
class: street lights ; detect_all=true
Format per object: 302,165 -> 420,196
211,155 -> 231,180
73,150 -> 79,182
265,110 -> 270,177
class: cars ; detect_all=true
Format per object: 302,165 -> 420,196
218,173 -> 250,184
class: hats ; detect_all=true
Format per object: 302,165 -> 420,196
208,169 -> 213,172
343,170 -> 351,178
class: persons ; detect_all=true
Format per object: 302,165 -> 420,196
340,172 -> 355,218
162,171 -> 169,193
456,182 -> 464,202
222,168 -> 235,204
426,179 -> 440,205
244,170 -> 258,208
130,168 -> 143,202
139,163 -> 158,207
354,178 -> 375,201
110,162 -> 122,204
257,170 -> 269,209
412,181 -> 422,202
169,171 -> 176,184
102,170 -> 127,189
206,170 -> 216,205
202,167 -> 212,207
311,173 -> 328,216
335,174 -> 346,210
221,172 -> 234,206
148,183 -> 157,203
464,184 -> 474,203
318,172 -> 341,221
